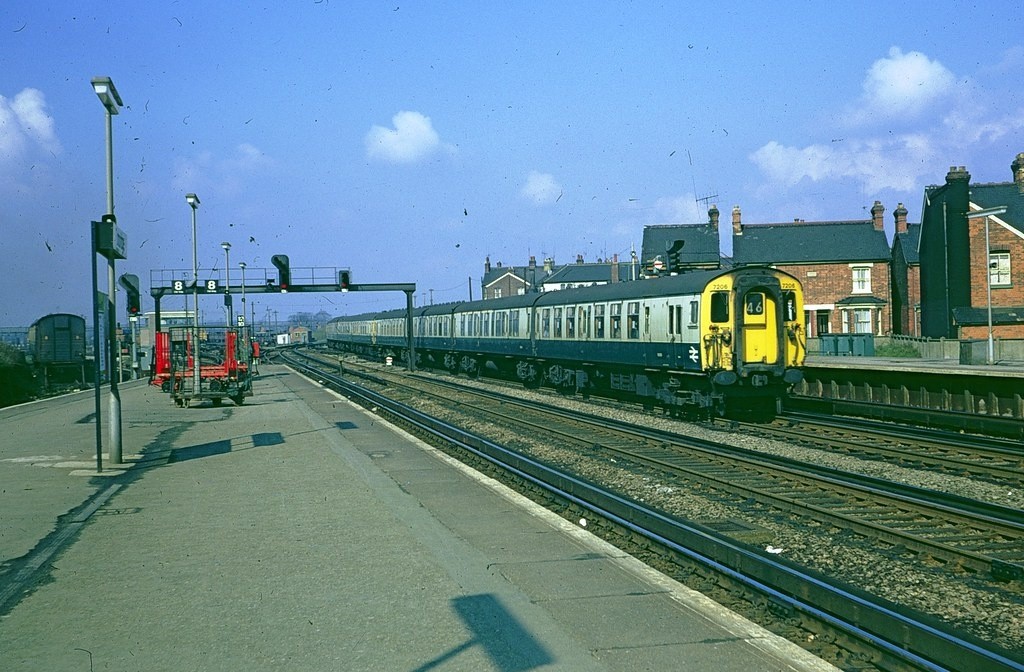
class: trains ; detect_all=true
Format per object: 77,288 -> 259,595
325,266 -> 807,423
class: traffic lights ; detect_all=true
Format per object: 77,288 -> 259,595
339,270 -> 349,291
118,274 -> 140,314
271,254 -> 290,290
